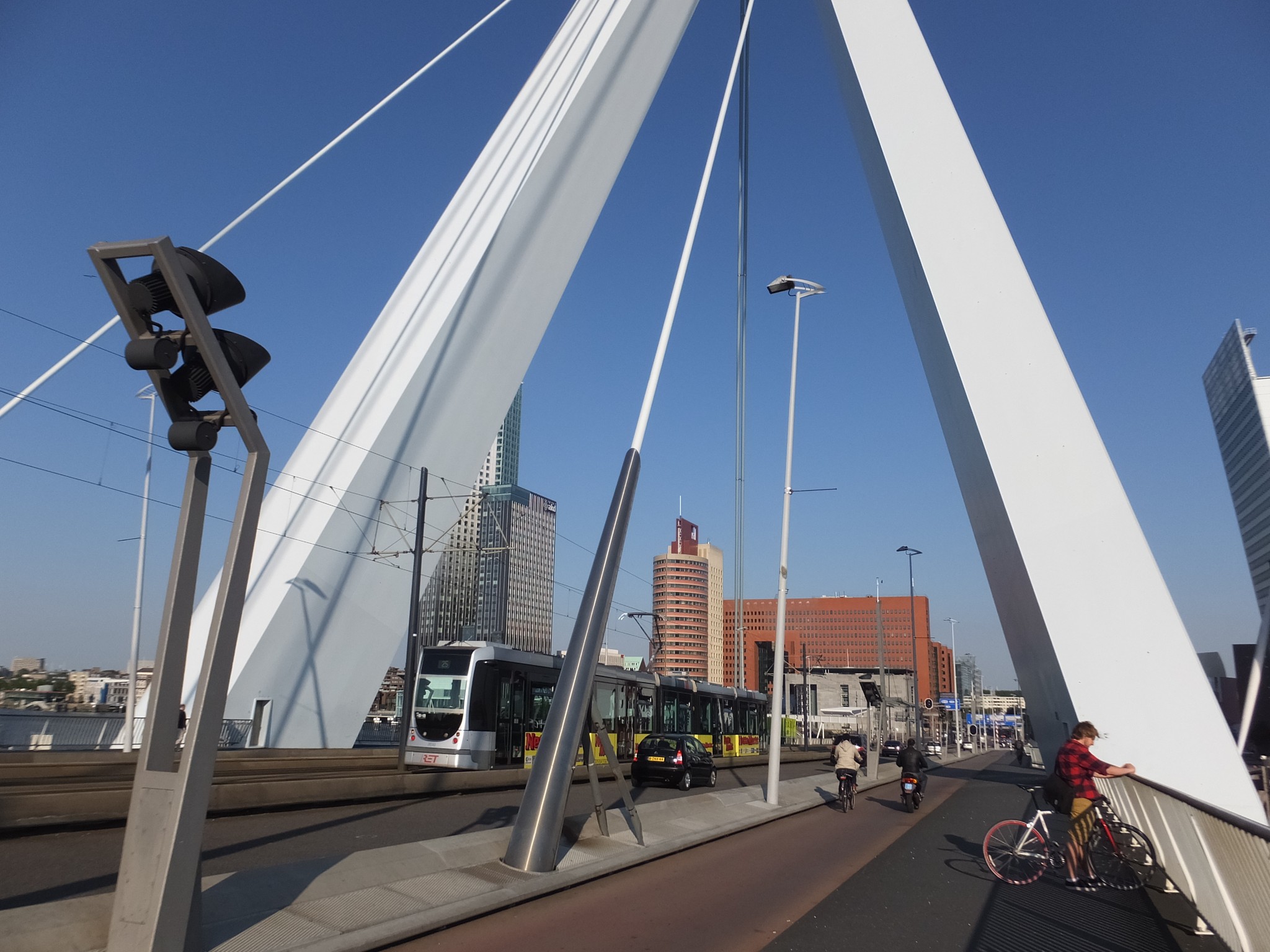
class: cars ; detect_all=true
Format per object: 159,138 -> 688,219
962,741 -> 972,751
630,733 -> 717,791
882,740 -> 905,757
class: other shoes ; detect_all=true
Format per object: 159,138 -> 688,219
1065,880 -> 1097,892
920,794 -> 925,798
900,794 -> 904,799
839,795 -> 843,801
1080,877 -> 1106,886
852,786 -> 858,793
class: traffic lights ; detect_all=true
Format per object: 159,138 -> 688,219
925,698 -> 933,709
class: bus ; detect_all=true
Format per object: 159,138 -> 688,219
998,725 -> 1021,748
402,637 -> 768,773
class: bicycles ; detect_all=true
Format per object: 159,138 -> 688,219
982,784 -> 1157,891
833,759 -> 863,813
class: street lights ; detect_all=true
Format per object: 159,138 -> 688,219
943,617 -> 961,758
86,235 -> 275,952
896,546 -> 923,753
859,679 -> 884,782
765,274 -> 827,805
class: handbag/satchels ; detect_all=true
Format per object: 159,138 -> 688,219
1041,758 -> 1089,814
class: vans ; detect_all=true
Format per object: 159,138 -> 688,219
924,741 -> 943,756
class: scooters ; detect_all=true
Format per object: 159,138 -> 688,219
900,764 -> 928,813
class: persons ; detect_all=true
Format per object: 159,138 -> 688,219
1015,736 -> 1024,765
834,734 -> 862,802
175,705 -> 187,752
1042,721 -> 1136,890
896,738 -> 928,801
